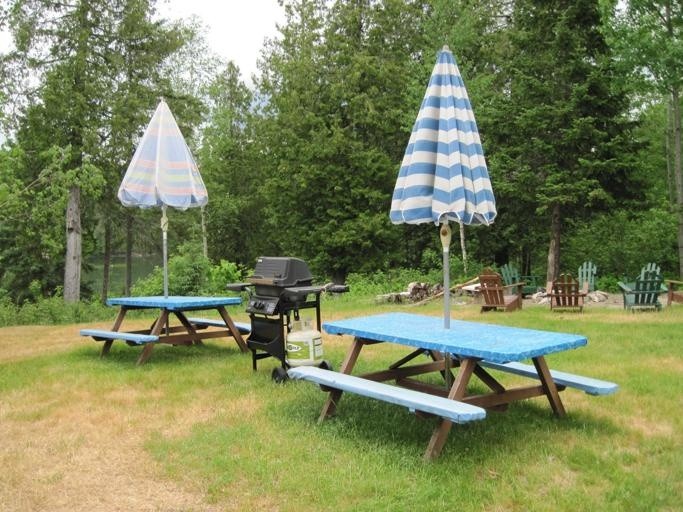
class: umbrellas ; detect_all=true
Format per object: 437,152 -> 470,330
389,45 -> 498,389
117,97 -> 210,340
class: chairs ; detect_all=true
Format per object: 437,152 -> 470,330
667,280 -> 683,305
479,267 -> 521,313
547,274 -> 587,312
576,261 -> 597,291
633,262 -> 661,290
500,262 -> 537,299
617,271 -> 668,313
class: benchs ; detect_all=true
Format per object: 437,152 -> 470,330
286,366 -> 486,424
80,329 -> 159,347
419,346 -> 618,396
188,317 -> 253,336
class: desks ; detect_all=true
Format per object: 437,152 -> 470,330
316,311 -> 588,466
99,295 -> 249,366
